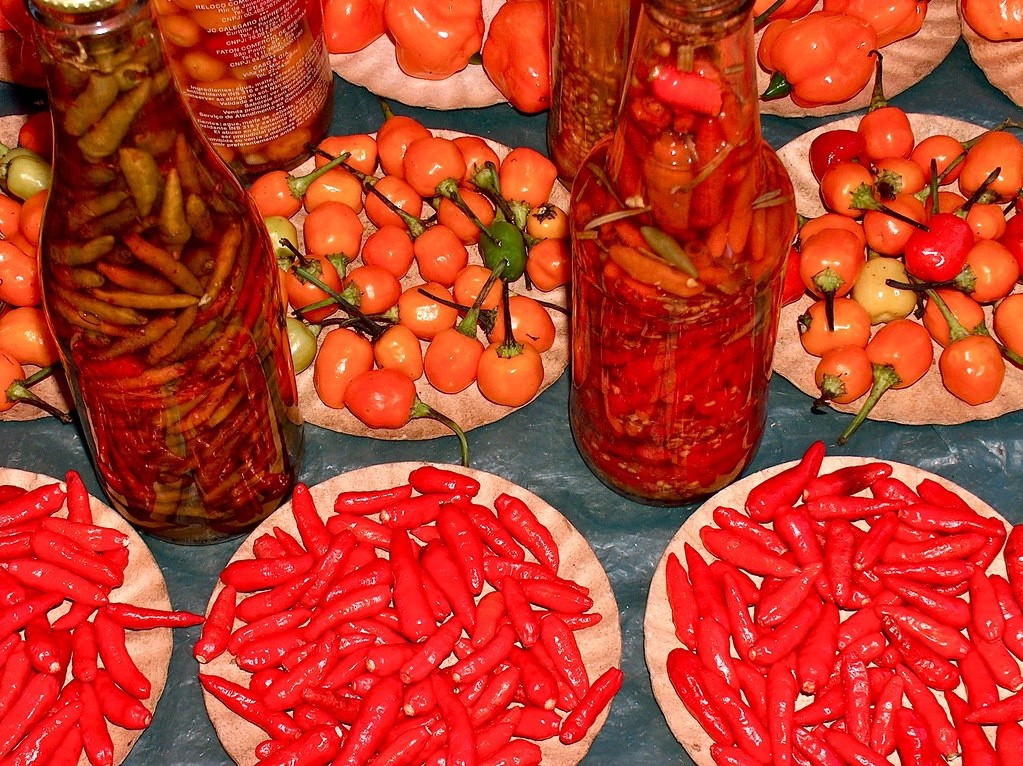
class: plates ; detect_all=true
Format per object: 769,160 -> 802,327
754,0 -> 960,117
330,0 -> 509,110
772,113 -> 1023,425
0,469 -> 173,766
956,0 -> 1023,108
288,127 -> 572,440
1,115 -> 71,421
644,455 -> 1023,764
200,460 -> 622,766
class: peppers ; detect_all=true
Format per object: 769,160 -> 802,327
744,0 -> 1023,444
0,0 -> 797,766
664,441 -> 1023,766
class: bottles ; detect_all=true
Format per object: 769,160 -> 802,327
547,0 -> 639,194
153,0 -> 333,186
24,0 -> 304,546
571,0 -> 797,508
